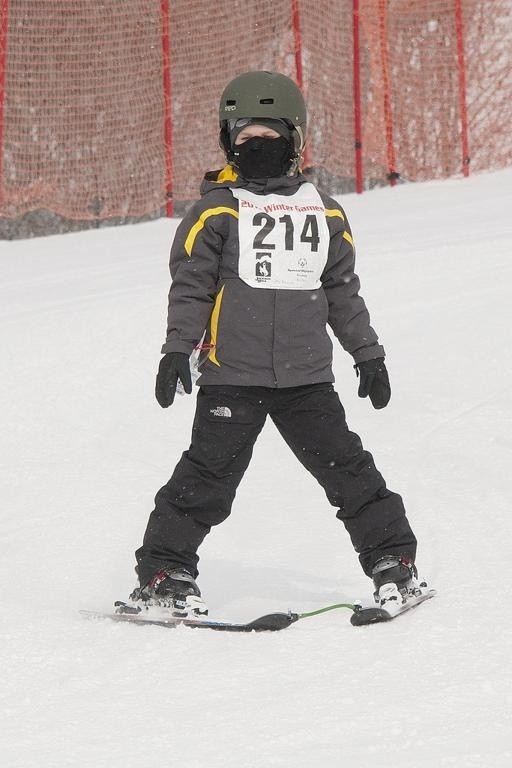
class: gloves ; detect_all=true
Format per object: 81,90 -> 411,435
353,357 -> 391,411
153,351 -> 194,410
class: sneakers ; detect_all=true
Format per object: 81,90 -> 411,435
128,565 -> 208,621
370,552 -> 419,607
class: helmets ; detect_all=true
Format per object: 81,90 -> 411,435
218,71 -> 308,178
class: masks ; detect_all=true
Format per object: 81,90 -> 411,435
233,135 -> 292,177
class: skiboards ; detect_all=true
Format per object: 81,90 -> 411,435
78,585 -> 437,631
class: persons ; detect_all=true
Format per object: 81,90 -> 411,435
114,71 -> 417,613
